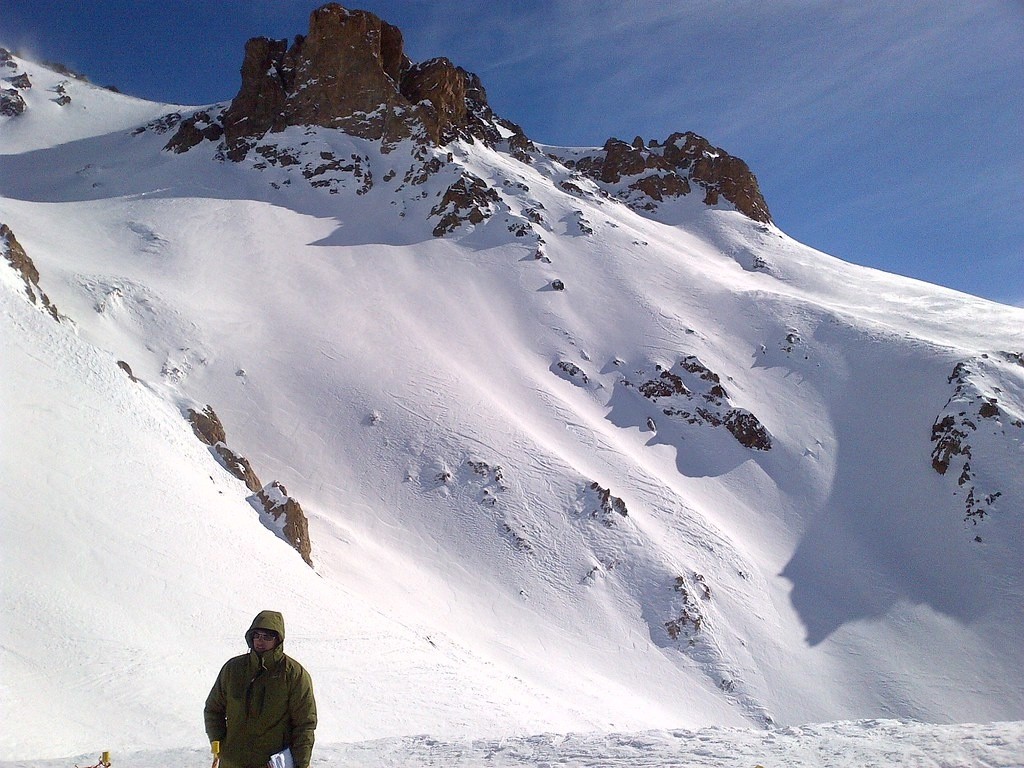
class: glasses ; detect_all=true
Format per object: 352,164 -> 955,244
252,632 -> 277,642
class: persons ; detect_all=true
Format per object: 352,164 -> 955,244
203,609 -> 318,768
